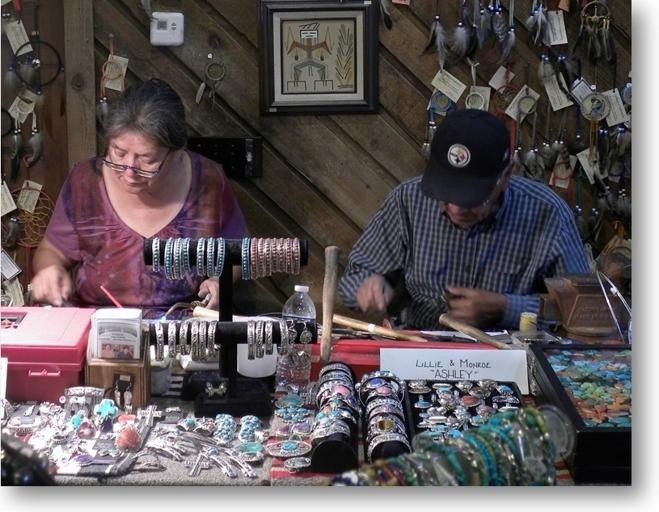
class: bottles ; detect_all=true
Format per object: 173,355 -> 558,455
274,283 -> 317,384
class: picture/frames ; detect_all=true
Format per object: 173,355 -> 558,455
257,1 -> 379,118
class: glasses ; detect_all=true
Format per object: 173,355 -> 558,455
102,148 -> 171,178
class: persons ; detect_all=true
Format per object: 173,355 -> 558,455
31,76 -> 252,312
337,109 -> 590,329
101,343 -> 116,359
118,346 -> 133,360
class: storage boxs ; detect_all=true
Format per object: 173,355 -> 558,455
1,307 -> 96,405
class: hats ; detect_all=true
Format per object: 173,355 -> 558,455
421,108 -> 510,208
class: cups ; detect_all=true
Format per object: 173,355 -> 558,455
520,312 -> 538,333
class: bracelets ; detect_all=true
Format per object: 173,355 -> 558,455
150,234 -> 302,282
309,363 -> 558,486
154,316 -> 293,361
